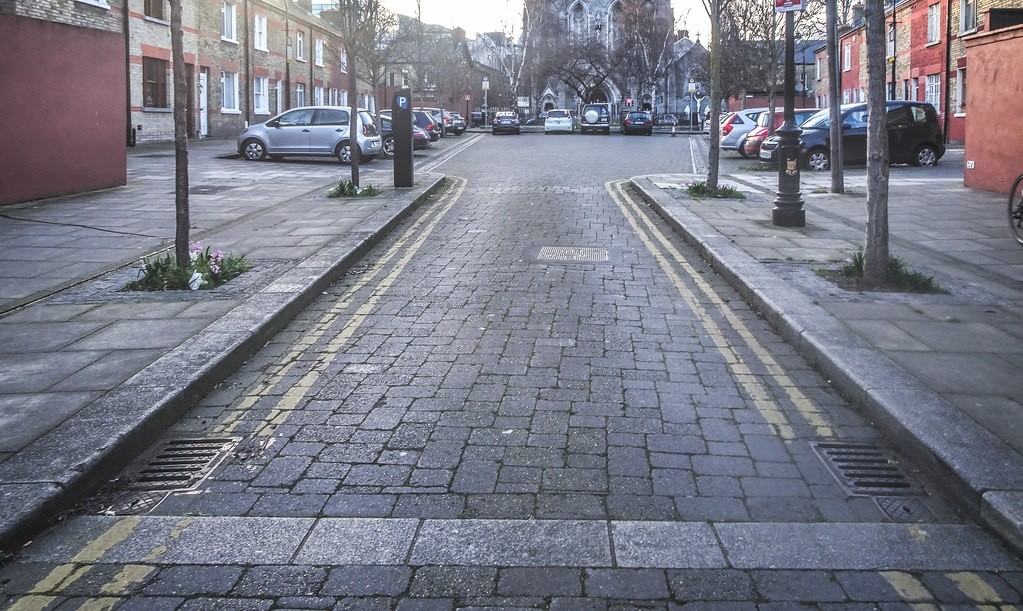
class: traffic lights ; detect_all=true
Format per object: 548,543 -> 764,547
661,96 -> 663,103
625,100 -> 627,106
631,100 -> 634,107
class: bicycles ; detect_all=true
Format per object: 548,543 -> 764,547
1007,174 -> 1023,245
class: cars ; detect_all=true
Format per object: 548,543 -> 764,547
744,107 -> 822,158
413,107 -> 454,135
448,111 -> 467,136
491,111 -> 520,135
657,114 -> 679,125
369,114 -> 430,159
701,107 -> 795,155
619,111 -> 653,136
236,106 -> 383,164
759,99 -> 947,172
526,113 -> 547,125
379,109 -> 441,142
545,109 -> 577,133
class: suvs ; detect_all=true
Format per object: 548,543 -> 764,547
580,103 -> 612,135
